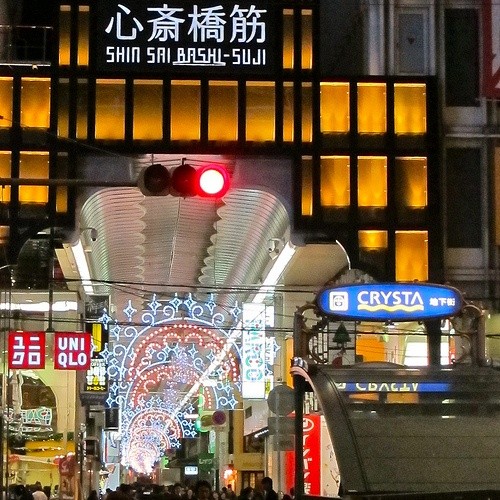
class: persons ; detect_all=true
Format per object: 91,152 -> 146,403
5,477 -> 299,499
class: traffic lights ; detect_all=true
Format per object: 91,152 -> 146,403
138,164 -> 229,199
